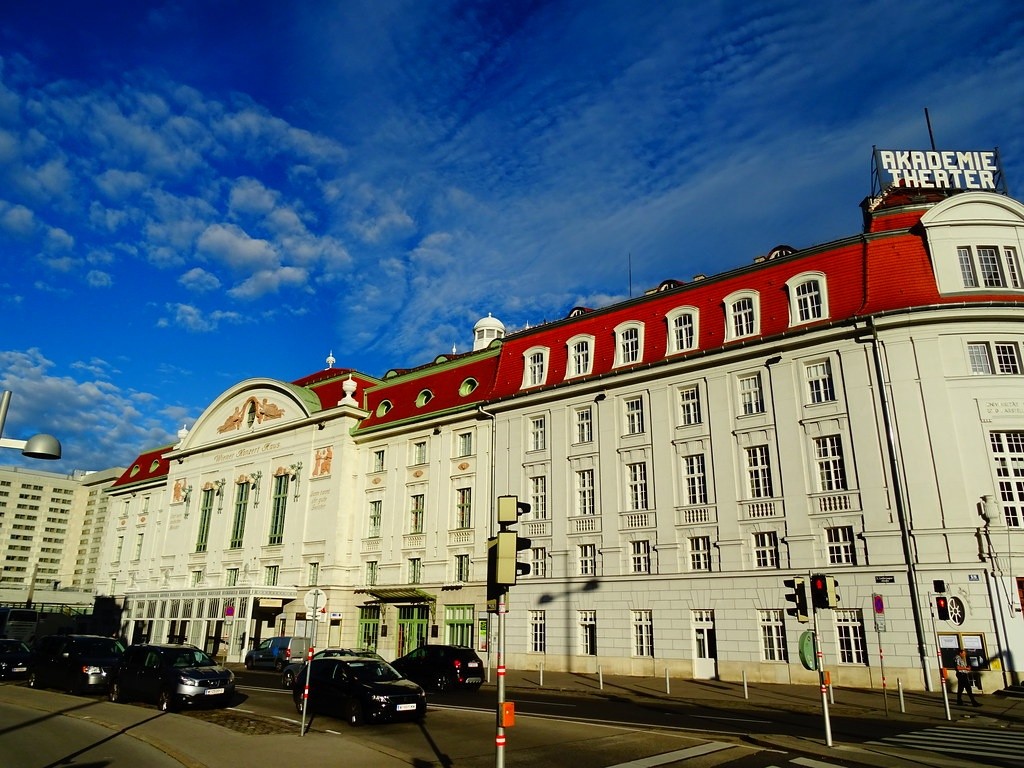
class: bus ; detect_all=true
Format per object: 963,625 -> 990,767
3,608 -> 41,642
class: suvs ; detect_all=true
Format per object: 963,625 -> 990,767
390,644 -> 485,690
281,649 -> 390,689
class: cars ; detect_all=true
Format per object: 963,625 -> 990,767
108,644 -> 235,711
27,634 -> 125,695
0,639 -> 30,677
245,637 -> 311,672
293,656 -> 427,724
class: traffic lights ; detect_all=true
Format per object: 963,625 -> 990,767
810,573 -> 840,609
496,495 -> 532,583
783,576 -> 810,623
936,596 -> 950,620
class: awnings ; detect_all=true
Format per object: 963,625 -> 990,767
354,588 -> 437,603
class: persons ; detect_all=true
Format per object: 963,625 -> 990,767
953,650 -> 983,707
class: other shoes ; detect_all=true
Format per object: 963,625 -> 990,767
972,702 -> 984,707
957,702 -> 968,706
